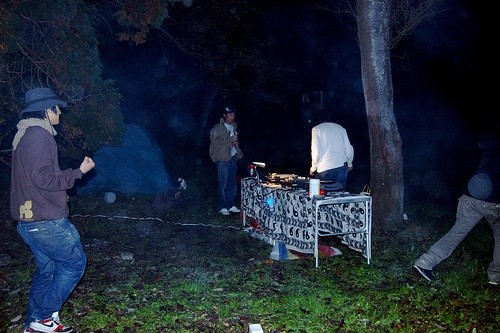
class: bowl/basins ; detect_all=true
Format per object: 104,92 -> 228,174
313,195 -> 325,199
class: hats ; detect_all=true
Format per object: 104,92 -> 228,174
21,88 -> 68,113
224,106 -> 238,114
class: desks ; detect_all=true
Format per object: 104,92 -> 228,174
241,177 -> 373,268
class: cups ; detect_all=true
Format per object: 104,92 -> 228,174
275,176 -> 280,184
309,179 -> 320,198
272,173 -> 277,180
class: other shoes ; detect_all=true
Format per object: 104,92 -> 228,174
219,208 -> 230,215
489,280 -> 500,285
413,265 -> 436,282
228,206 -> 240,213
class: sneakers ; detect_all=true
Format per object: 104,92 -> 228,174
23,311 -> 74,333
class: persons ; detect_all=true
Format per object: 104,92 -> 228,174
11,87 -> 95,333
304,108 -> 354,191
412,124 -> 500,285
209,107 -> 243,215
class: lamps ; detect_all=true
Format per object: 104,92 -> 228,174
253,162 -> 265,184
